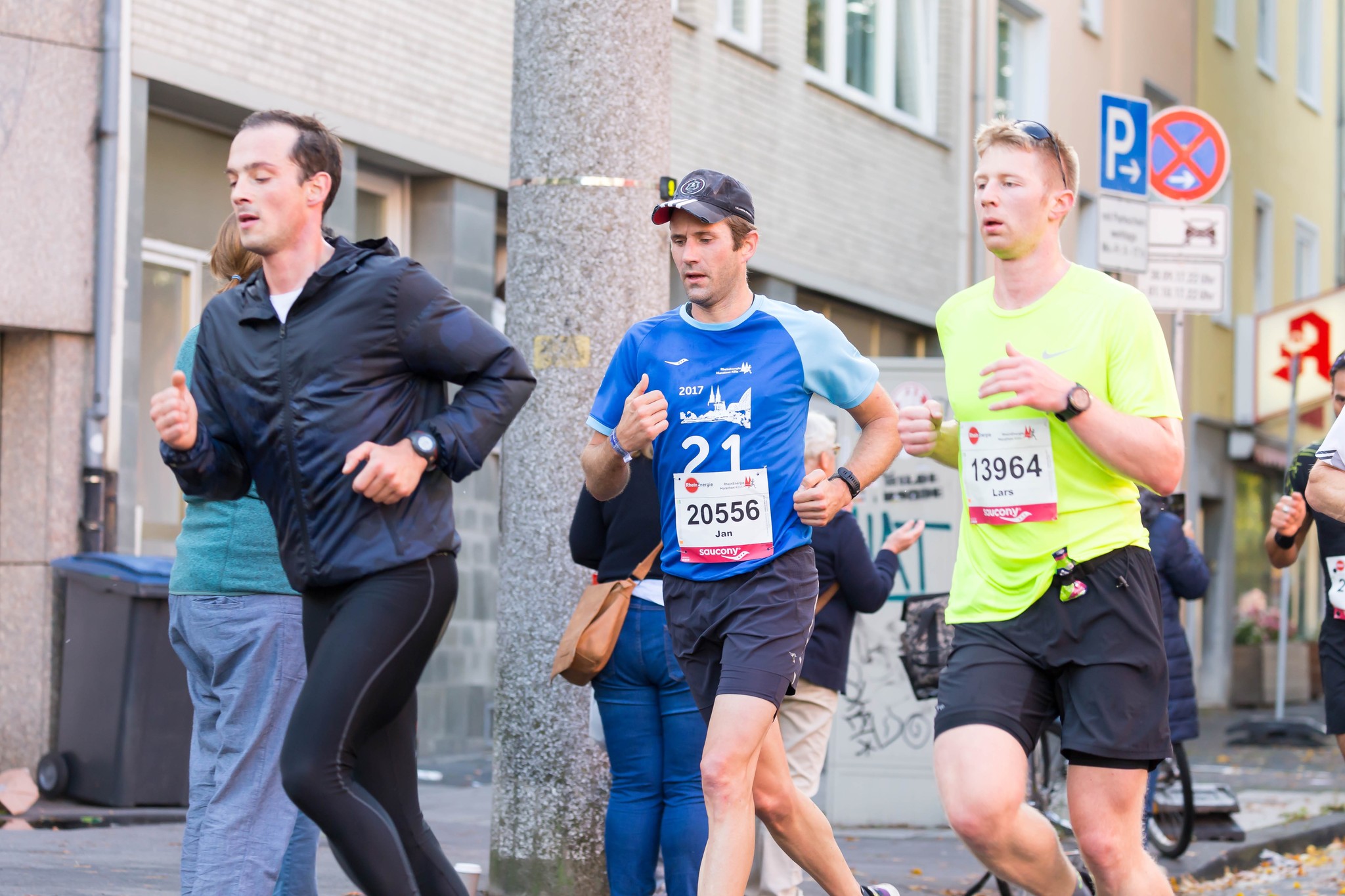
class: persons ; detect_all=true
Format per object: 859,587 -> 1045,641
591,442 -> 708,896
751,411 -> 923,896
1140,488 -> 1208,843
901,117 -> 1188,896
150,111 -> 534,896
582,167 -> 900,896
1266,353 -> 1345,775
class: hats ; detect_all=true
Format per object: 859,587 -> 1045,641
651,169 -> 755,225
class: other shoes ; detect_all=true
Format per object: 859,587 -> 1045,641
860,882 -> 900,896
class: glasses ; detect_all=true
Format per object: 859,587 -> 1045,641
1013,120 -> 1067,190
833,447 -> 841,457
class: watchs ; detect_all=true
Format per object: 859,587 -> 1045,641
407,430 -> 439,473
1054,381 -> 1091,422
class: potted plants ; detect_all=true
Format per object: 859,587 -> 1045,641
1231,625 -> 1311,707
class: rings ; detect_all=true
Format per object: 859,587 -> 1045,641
1282,506 -> 1290,513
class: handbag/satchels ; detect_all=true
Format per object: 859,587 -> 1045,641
901,595 -> 955,698
549,578 -> 637,687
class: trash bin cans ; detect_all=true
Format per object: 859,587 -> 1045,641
46,550 -> 192,805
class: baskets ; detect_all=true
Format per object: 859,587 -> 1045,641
898,646 -> 953,700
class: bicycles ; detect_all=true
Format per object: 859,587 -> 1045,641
901,592 -> 1189,853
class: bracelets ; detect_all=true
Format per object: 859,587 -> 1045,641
1274,529 -> 1299,549
609,425 -> 632,463
828,467 -> 861,500
